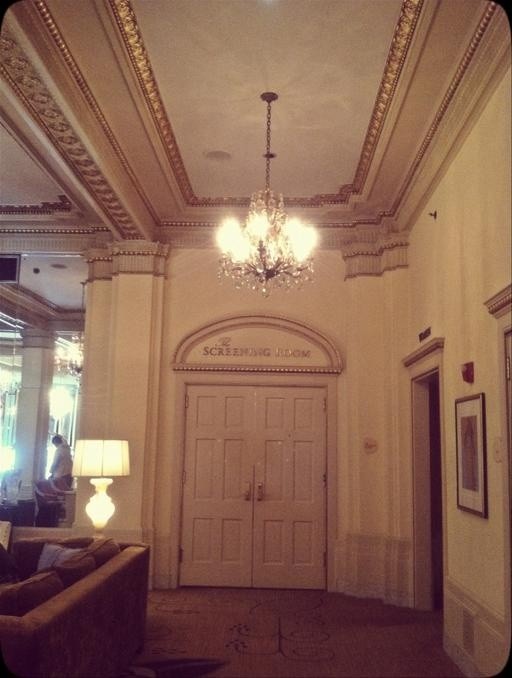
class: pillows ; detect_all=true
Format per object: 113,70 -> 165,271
34,541 -> 81,570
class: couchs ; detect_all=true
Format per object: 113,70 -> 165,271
1,537 -> 151,676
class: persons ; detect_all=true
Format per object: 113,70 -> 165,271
49,435 -> 73,490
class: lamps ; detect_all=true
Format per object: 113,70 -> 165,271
69,438 -> 133,541
209,89 -> 321,295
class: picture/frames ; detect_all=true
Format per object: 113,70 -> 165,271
454,391 -> 490,520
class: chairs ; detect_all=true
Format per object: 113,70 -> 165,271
0,468 -> 73,526
